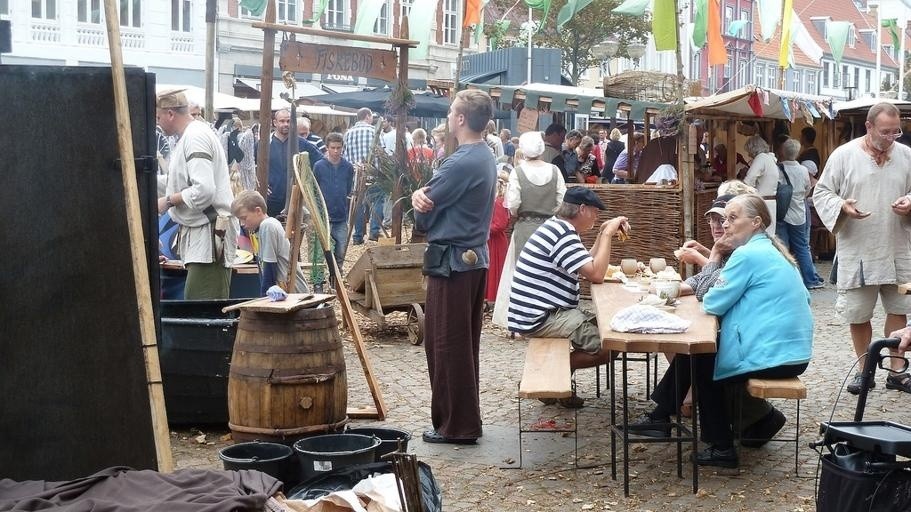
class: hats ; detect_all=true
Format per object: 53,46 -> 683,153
564,187 -> 607,212
704,194 -> 736,218
520,131 -> 546,158
158,88 -> 188,108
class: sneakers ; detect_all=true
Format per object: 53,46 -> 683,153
617,413 -> 672,444
518,383 -> 584,407
808,281 -> 824,288
741,408 -> 785,448
690,446 -> 739,468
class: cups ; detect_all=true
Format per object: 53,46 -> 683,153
621,258 -> 681,306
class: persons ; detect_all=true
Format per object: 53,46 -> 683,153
155,88 -> 911,468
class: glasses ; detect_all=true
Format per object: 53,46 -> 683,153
706,217 -> 725,224
876,127 -> 903,139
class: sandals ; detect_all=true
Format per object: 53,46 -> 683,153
847,371 -> 876,393
886,372 -> 911,392
423,429 -> 477,444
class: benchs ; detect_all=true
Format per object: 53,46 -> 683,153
516,338 -> 576,467
736,373 -> 807,475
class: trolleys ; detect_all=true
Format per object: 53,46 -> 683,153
327,243 -> 427,346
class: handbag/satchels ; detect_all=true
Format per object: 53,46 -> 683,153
816,454 -> 894,512
832,443 -> 893,471
777,185 -> 793,220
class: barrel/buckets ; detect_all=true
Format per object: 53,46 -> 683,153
219,442 -> 291,479
294,435 -> 381,482
343,425 -> 413,469
156,305 -> 241,434
228,304 -> 348,447
